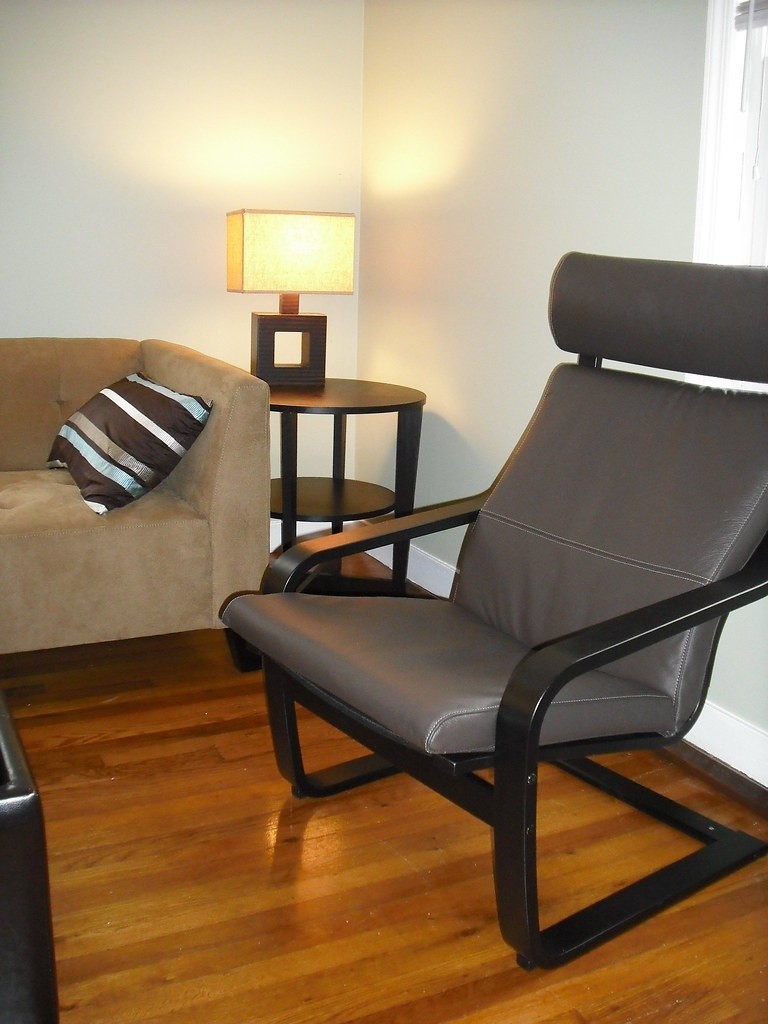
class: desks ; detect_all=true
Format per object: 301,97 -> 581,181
260,374 -> 427,590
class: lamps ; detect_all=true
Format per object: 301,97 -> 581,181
223,207 -> 355,387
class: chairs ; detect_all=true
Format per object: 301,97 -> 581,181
216,249 -> 768,972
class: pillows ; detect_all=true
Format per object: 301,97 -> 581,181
45,368 -> 212,516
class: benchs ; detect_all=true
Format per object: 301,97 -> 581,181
0,336 -> 271,673
